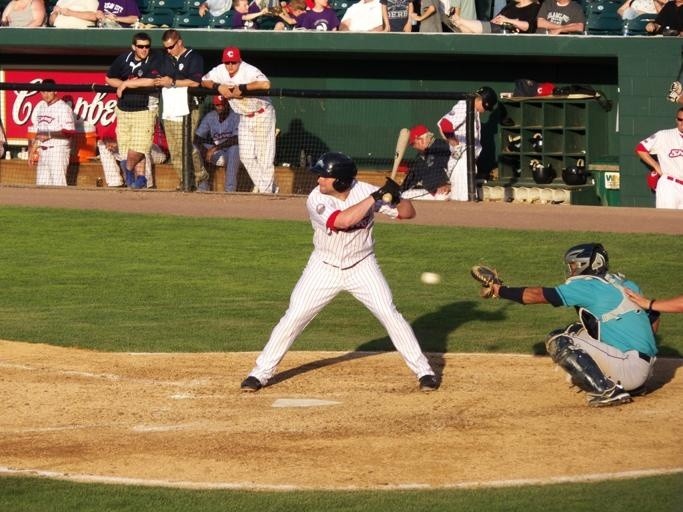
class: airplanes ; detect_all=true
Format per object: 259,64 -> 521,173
470,263 -> 502,298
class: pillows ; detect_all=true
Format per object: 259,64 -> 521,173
561,159 -> 586,184
528,160 -> 552,184
474,86 -> 498,112
310,151 -> 358,193
563,242 -> 608,280
507,135 -> 521,153
528,133 -> 542,151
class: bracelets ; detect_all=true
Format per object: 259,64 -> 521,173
239,84 -> 247,92
649,299 -> 656,309
212,84 -> 220,89
499,286 -> 528,304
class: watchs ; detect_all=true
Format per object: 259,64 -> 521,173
171,79 -> 176,87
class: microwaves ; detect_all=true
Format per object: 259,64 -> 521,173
381,128 -> 410,201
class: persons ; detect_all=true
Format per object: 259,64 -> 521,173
194,95 -> 240,192
105,33 -> 163,190
635,109 -> 682,207
436,87 -> 497,202
491,243 -> 661,407
0,0 -> 683,37
151,30 -> 209,192
201,46 -> 281,194
622,287 -> 683,314
102,115 -> 170,188
397,125 -> 455,201
238,151 -> 441,395
27,79 -> 74,186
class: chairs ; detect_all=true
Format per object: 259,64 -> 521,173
131,176 -> 148,189
241,377 -> 261,391
418,375 -> 438,391
120,160 -> 135,188
198,179 -> 211,191
587,383 -> 630,407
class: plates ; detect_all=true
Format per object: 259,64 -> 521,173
495,99 -> 609,191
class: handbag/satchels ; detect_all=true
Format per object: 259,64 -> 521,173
649,299 -> 656,309
499,286 -> 528,304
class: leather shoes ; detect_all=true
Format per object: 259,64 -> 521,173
384,176 -> 402,205
371,184 -> 401,201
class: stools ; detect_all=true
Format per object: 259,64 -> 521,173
246,109 -> 264,117
638,350 -> 649,364
667,177 -> 682,184
41,146 -> 47,150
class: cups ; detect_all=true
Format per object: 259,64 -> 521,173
135,44 -> 150,48
165,41 -> 178,50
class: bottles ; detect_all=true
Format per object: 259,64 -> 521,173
406,124 -> 428,145
536,82 -> 554,96
647,171 -> 659,191
212,94 -> 226,105
222,46 -> 241,63
